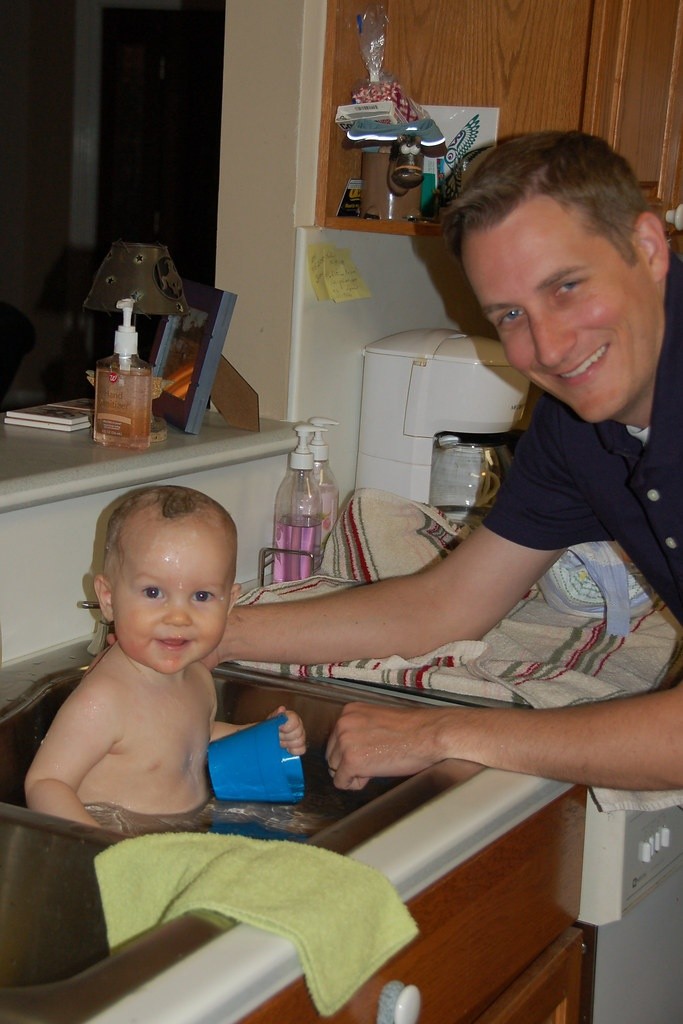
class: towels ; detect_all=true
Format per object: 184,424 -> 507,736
227,487 -> 683,712
92,830 -> 422,1020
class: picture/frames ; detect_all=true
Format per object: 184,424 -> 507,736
149,278 -> 259,435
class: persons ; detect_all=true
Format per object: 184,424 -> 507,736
106,132 -> 683,792
25,486 -> 307,825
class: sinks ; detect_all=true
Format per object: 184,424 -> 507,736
0,671 -> 450,844
0,816 -> 191,992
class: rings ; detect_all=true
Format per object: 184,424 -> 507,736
328,761 -> 337,772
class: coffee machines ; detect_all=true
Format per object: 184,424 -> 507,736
354,329 -> 533,535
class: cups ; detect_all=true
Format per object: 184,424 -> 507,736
209,710 -> 310,804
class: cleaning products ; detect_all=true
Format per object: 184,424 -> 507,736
271,417 -> 342,585
93,297 -> 153,450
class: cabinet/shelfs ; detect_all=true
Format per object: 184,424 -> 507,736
316,1 -> 683,249
236,782 -> 587,1023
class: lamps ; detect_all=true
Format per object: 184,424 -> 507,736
83,242 -> 191,443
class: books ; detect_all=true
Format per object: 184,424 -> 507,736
4,399 -> 97,433
